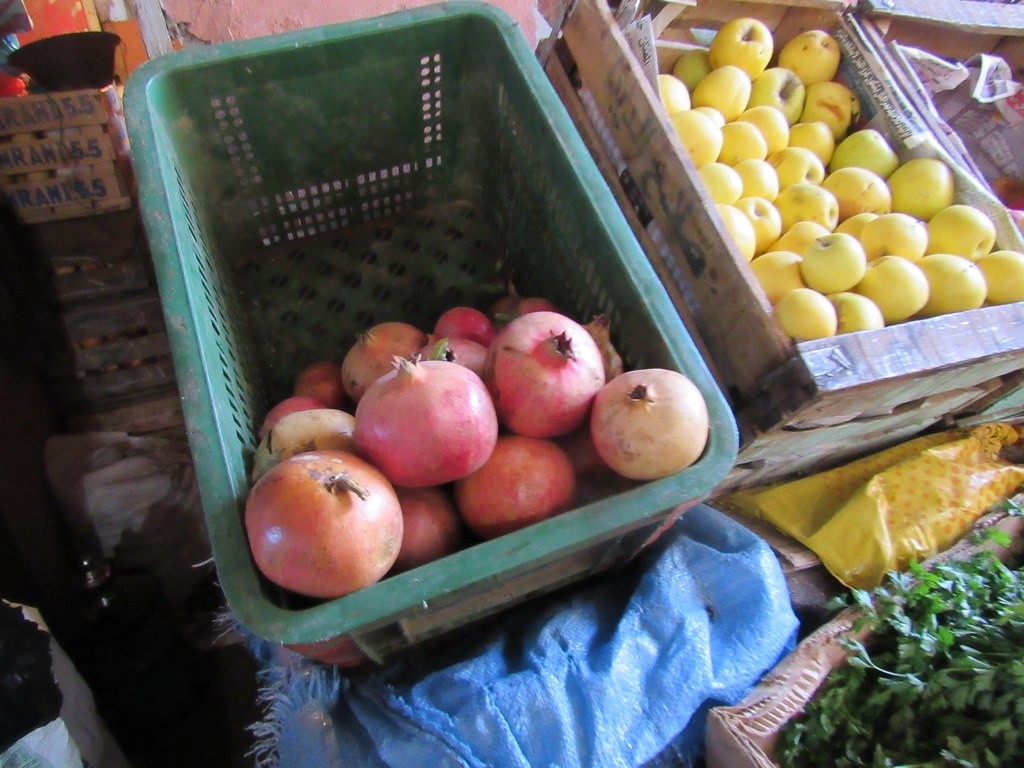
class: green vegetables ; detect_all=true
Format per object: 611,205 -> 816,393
772,496 -> 1024,768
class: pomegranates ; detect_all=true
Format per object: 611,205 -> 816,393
243,290 -> 709,599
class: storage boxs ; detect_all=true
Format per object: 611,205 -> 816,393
852,0 -> 1024,431
534,0 -> 1024,499
121,0 -> 741,665
0,81 -> 138,225
703,492 -> 1024,768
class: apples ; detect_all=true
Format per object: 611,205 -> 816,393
657,18 -> 1024,343
